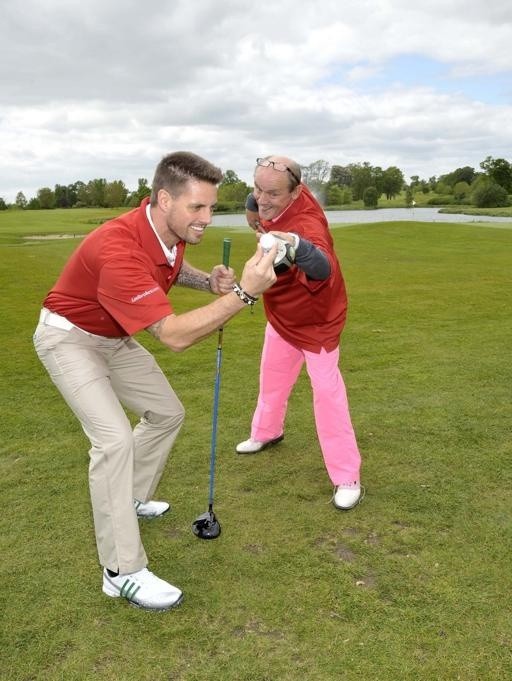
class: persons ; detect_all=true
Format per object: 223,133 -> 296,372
32,151 -> 278,611
245,154 -> 362,511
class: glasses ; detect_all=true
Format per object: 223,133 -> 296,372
257,159 -> 301,184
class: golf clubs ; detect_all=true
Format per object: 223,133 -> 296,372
192,239 -> 230,539
256,220 -> 296,274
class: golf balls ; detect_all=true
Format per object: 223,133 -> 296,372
260,233 -> 276,249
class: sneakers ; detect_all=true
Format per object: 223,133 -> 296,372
236,429 -> 284,455
102,564 -> 185,612
134,499 -> 170,518
333,480 -> 361,510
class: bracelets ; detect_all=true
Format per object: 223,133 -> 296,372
233,284 -> 259,305
205,274 -> 211,289
